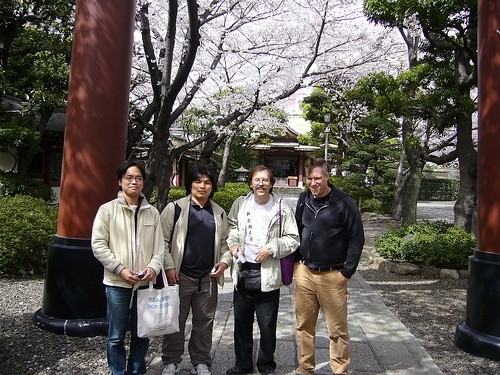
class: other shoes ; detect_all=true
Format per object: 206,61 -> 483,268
285,370 -> 299,375
226,366 -> 252,375
258,369 -> 277,375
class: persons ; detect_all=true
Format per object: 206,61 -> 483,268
90,162 -> 165,375
160,166 -> 233,375
227,165 -> 301,375
286,157 -> 364,375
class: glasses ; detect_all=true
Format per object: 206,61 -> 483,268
123,176 -> 142,182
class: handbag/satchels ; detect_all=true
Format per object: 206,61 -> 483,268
135,259 -> 179,337
280,252 -> 293,285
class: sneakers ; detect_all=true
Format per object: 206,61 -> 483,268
191,364 -> 211,375
162,363 -> 181,375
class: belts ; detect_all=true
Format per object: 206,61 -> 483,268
300,258 -> 344,271
